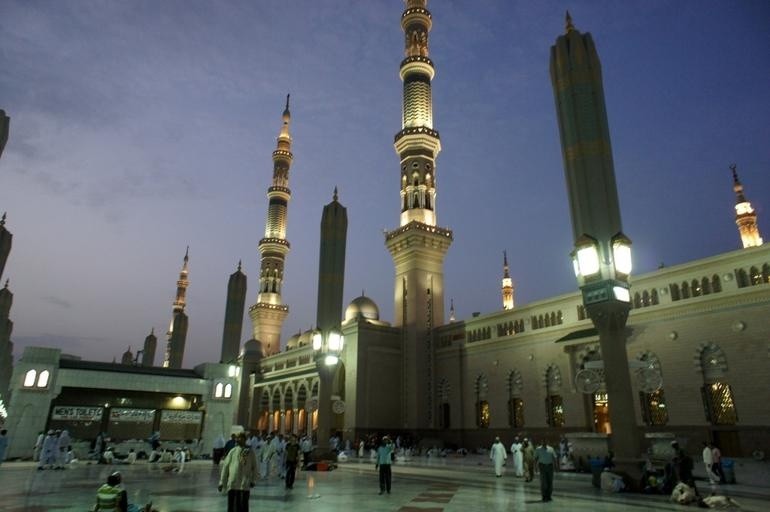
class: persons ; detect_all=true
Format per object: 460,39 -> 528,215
489,434 -> 567,501
376,436 -> 394,494
701,441 -> 720,485
218,428 -> 311,511
331,434 -> 375,457
600,451 -> 660,494
396,436 -> 447,457
669,442 -> 689,482
33,427 -> 190,512
0,429 -> 7,464
